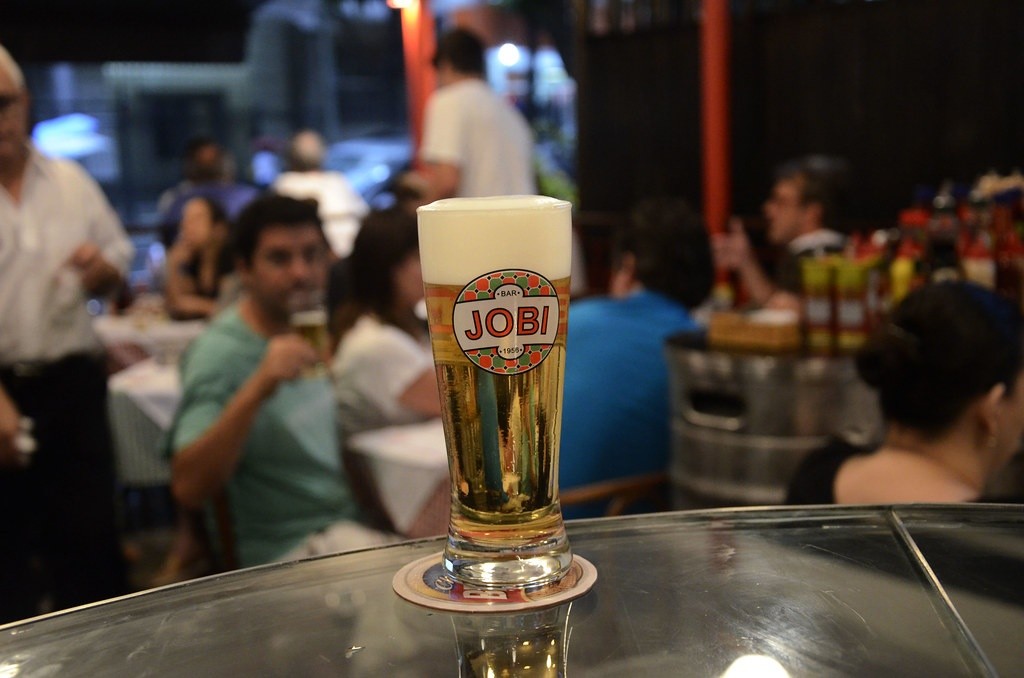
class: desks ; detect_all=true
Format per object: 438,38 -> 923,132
349,422 -> 453,534
0,477 -> 1024,677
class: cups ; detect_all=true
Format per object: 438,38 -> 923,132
415,201 -> 572,590
447,613 -> 571,678
287,294 -> 331,380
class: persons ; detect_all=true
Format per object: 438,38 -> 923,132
94,25 -> 864,571
784,271 -> 1024,513
0,41 -> 136,625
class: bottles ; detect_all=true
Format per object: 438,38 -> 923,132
789,188 -> 1024,351
